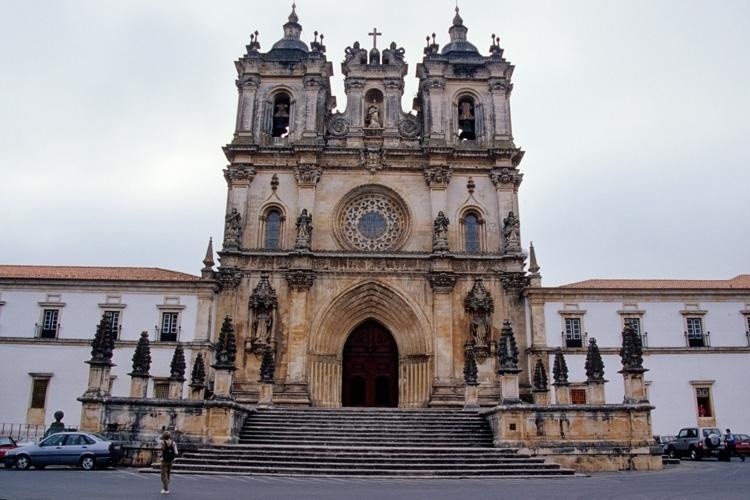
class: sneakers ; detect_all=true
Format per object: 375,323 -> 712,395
160,489 -> 169,494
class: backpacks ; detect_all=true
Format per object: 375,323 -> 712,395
163,441 -> 174,463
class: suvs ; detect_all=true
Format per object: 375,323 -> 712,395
650,427 -> 728,463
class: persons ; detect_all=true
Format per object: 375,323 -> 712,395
293,208 -> 312,250
431,210 -> 452,250
722,428 -> 746,462
502,210 -> 520,251
224,207 -> 242,240
158,430 -> 179,494
698,404 -> 709,418
368,98 -> 382,127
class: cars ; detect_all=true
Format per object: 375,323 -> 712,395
719,432 -> 750,456
0,435 -> 18,468
3,432 -> 128,472
